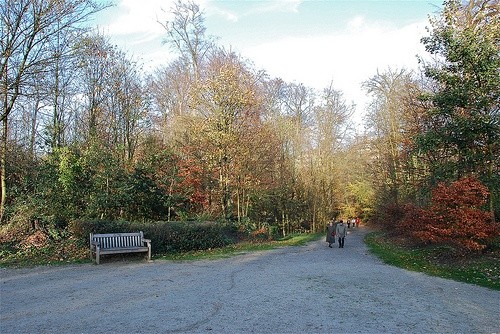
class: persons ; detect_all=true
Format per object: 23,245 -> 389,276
352,218 -> 355,228
347,219 -> 351,228
356,217 -> 360,227
336,221 -> 347,248
326,221 -> 336,248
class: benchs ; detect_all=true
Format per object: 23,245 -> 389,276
89,231 -> 151,264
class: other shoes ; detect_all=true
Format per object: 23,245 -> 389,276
342,245 -> 343,248
329,245 -> 332,248
339,246 -> 341,248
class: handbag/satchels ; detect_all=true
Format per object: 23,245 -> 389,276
331,231 -> 336,236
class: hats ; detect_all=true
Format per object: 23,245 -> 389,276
338,221 -> 343,223
